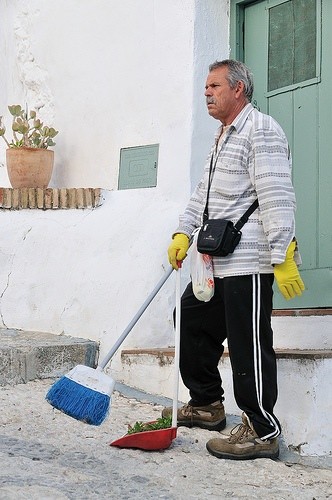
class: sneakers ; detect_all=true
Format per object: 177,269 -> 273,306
162,400 -> 226,431
206,412 -> 279,460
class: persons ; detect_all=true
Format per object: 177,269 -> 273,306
161,57 -> 306,461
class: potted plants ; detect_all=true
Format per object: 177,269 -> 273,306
0,101 -> 59,189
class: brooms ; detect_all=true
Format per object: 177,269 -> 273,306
43,259 -> 181,427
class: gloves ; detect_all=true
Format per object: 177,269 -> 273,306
273,241 -> 305,301
168,233 -> 190,270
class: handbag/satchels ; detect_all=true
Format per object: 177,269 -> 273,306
197,219 -> 242,257
